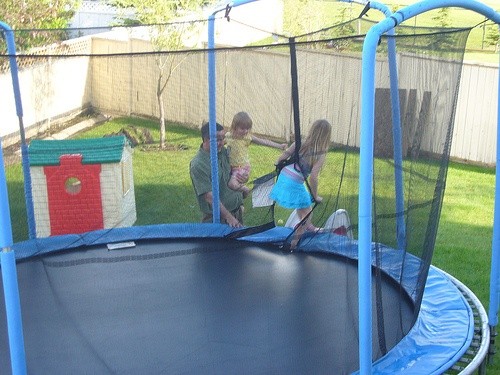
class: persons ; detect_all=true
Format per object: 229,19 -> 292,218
189,122 -> 251,229
269,118 -> 332,252
223,110 -> 288,199
